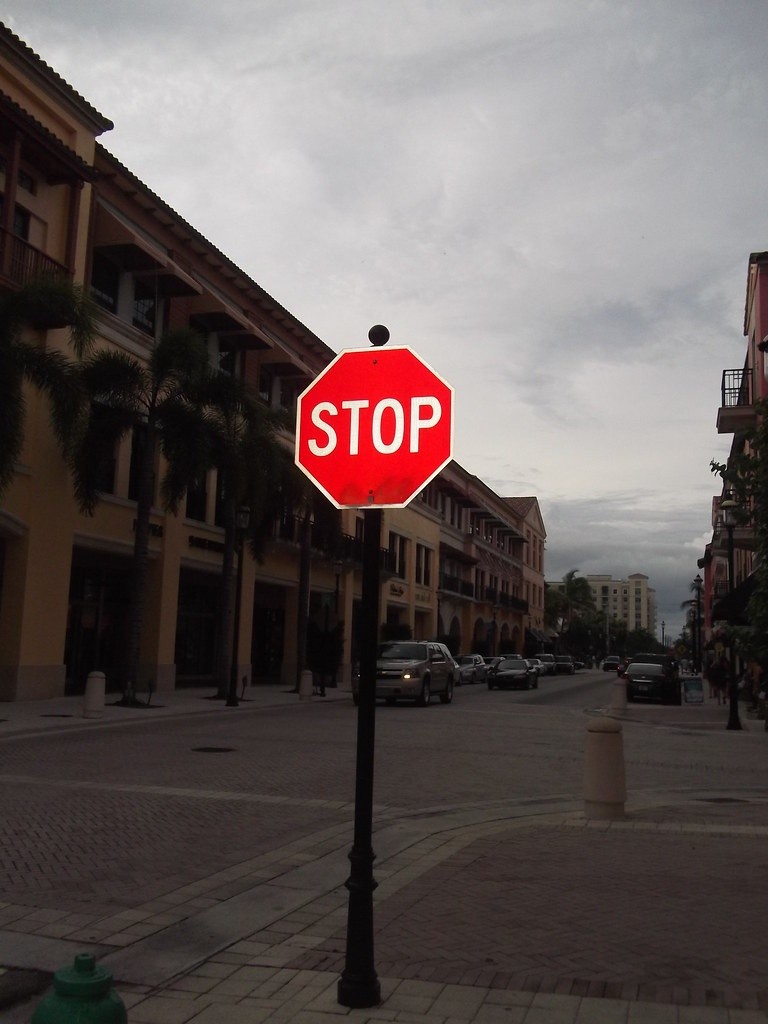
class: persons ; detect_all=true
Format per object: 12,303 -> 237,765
595,656 -> 601,672
743,657 -> 764,713
681,654 -> 730,705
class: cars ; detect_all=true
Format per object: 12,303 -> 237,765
452,654 -> 487,684
487,660 -> 538,690
528,659 -> 545,677
500,654 -> 522,660
617,657 -> 635,677
483,657 -> 505,672
555,656 -> 584,674
604,655 -> 620,671
624,662 -> 670,704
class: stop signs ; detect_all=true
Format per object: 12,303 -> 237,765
294,345 -> 454,510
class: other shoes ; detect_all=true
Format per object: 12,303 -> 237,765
723,697 -> 726,704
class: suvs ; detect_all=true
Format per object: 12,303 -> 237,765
638,653 -> 678,685
536,654 -> 558,674
352,640 -> 454,706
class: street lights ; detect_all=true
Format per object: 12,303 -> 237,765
331,557 -> 533,688
691,602 -> 697,674
661,621 -> 666,647
682,626 -> 686,638
694,575 -> 703,675
720,496 -> 745,730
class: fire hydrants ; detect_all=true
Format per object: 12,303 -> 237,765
33,952 -> 129,1024
